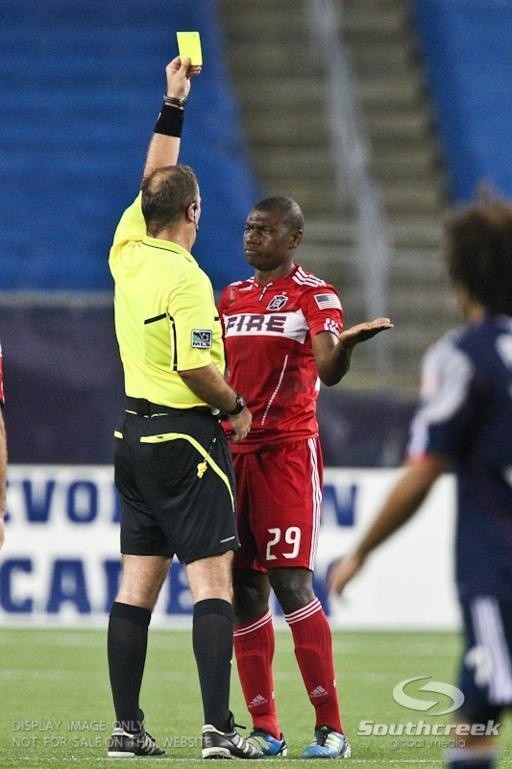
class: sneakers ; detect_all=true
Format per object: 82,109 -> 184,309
106,726 -> 167,757
301,724 -> 352,759
244,729 -> 288,755
200,711 -> 265,758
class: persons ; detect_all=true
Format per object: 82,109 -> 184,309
0,345 -> 9,548
107,54 -> 258,759
216,195 -> 393,757
326,186 -> 512,769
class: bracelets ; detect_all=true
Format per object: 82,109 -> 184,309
163,92 -> 189,107
152,104 -> 184,136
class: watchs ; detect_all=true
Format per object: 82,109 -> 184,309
228,395 -> 247,416
